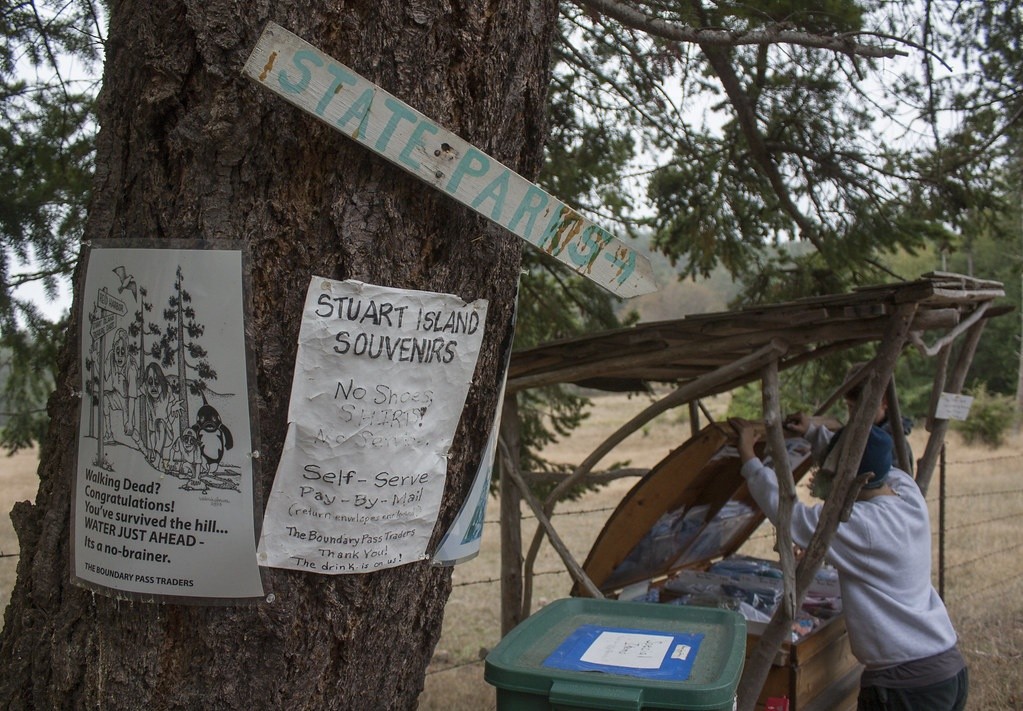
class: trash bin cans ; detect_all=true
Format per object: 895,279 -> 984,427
485,598 -> 748,711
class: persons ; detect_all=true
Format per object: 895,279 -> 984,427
789,361 -> 914,476
726,412 -> 970,711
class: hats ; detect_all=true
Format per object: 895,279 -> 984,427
831,425 -> 896,490
